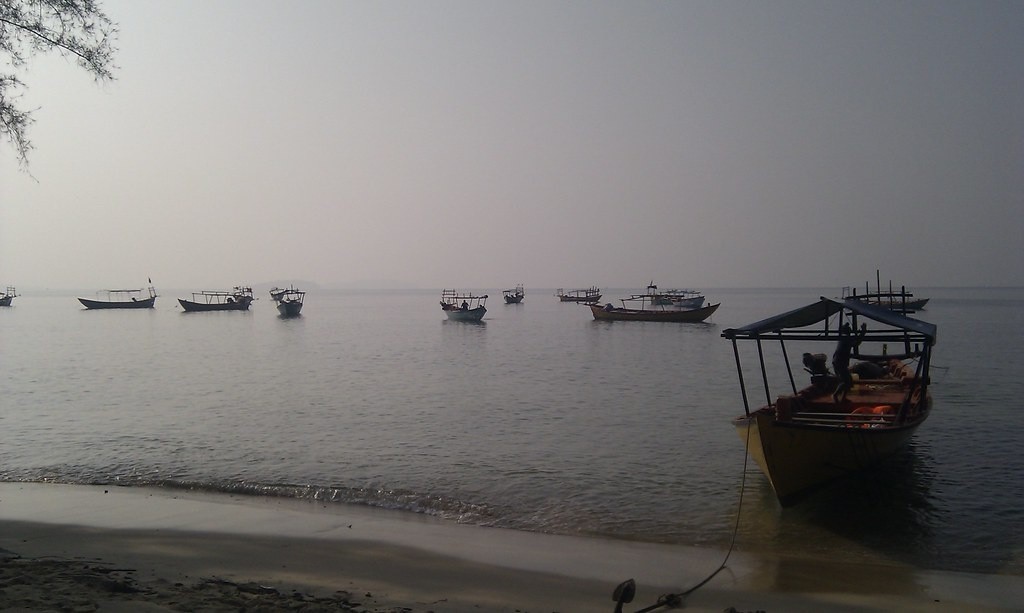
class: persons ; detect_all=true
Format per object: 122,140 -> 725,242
832,322 -> 867,402
605,303 -> 613,311
461,300 -> 469,311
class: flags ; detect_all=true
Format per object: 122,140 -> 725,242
149,278 -> 151,283
648,285 -> 657,289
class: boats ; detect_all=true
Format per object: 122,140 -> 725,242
269,284 -> 306,316
720,297 -> 939,510
584,282 -> 720,322
835,269 -> 930,309
0,292 -> 12,306
177,287 -> 254,310
77,279 -> 157,309
441,289 -> 488,321
502,285 -> 523,303
560,286 -> 601,302
652,288 -> 706,308
